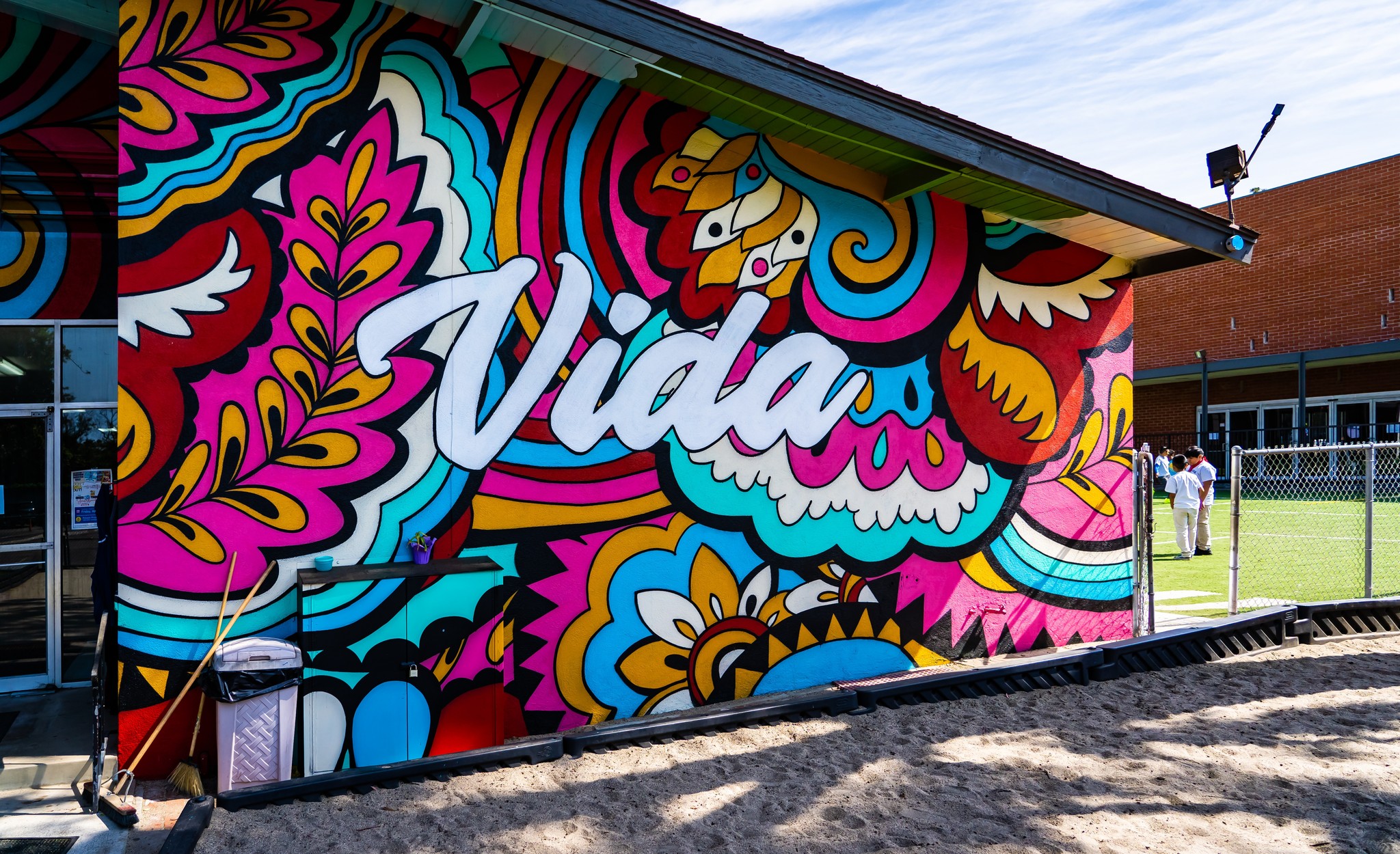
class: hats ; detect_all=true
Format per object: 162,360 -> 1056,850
1160,445 -> 1168,450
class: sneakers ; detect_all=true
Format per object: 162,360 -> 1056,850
1194,545 -> 1213,556
1165,498 -> 1170,503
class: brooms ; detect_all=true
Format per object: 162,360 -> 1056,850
84,559 -> 278,827
167,549 -> 239,799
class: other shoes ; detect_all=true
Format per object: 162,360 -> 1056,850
1178,554 -> 1193,558
1173,556 -> 1191,560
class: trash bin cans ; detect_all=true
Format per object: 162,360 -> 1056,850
212,636 -> 303,794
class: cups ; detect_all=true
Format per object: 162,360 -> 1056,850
314,556 -> 334,571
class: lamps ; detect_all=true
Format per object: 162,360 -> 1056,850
0,357 -> 26,377
1207,142 -> 1249,223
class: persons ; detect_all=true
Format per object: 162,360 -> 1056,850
1133,447 -> 1189,504
1184,446 -> 1216,555
1165,455 -> 1204,560
